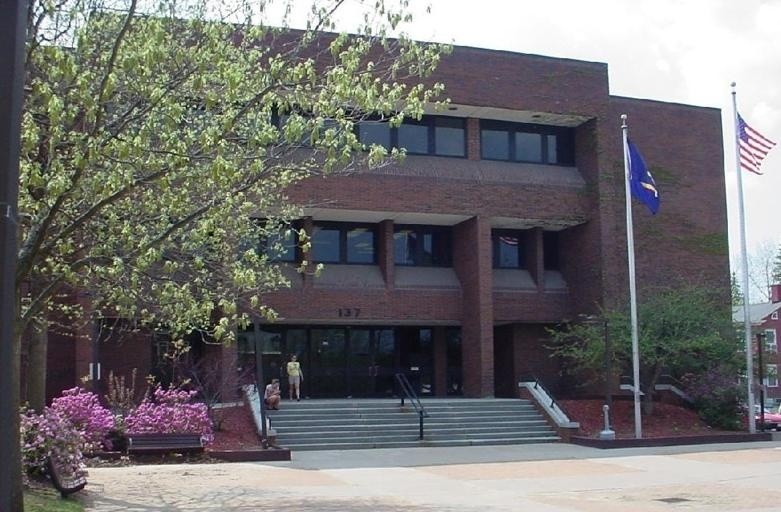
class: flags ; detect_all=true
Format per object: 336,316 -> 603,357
735,113 -> 777,175
622,133 -> 660,214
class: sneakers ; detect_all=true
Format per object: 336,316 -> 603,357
267,406 -> 279,410
297,398 -> 300,402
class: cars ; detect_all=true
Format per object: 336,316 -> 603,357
740,404 -> 781,432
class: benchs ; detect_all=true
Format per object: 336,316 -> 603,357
127,433 -> 205,464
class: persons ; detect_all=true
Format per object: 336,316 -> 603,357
286,354 -> 304,401
264,378 -> 282,410
